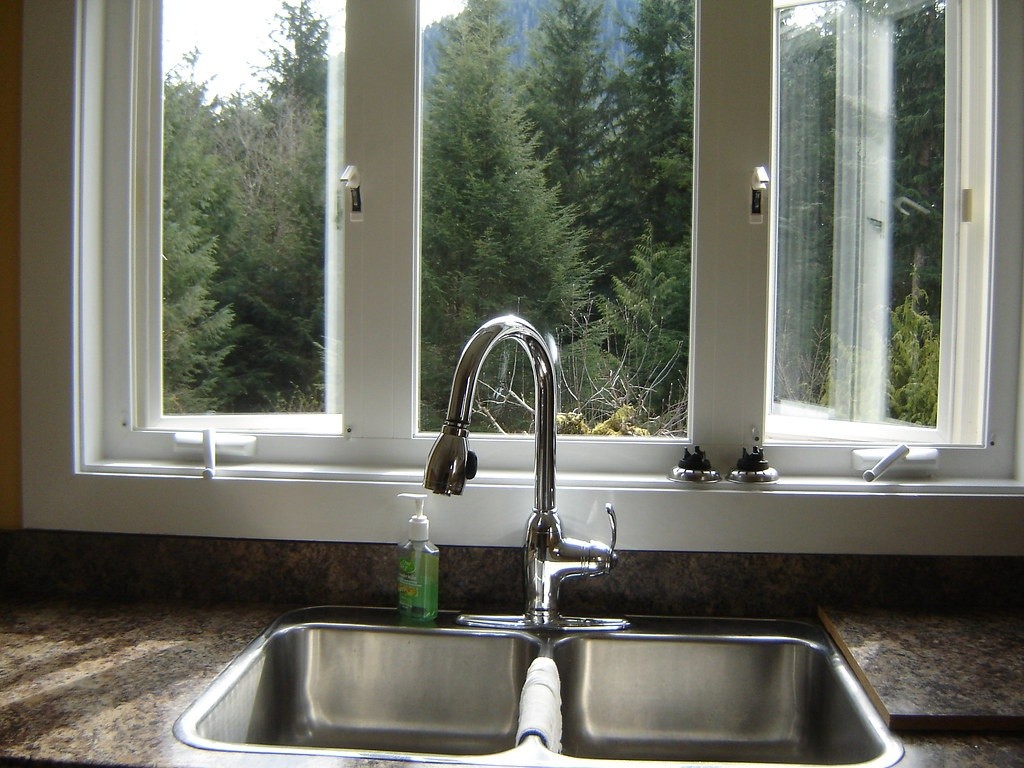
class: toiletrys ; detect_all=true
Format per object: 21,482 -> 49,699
397,490 -> 441,624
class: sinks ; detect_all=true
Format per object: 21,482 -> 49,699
539,604 -> 906,768
172,596 -> 541,768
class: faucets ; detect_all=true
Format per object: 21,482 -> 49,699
422,314 -> 620,634
